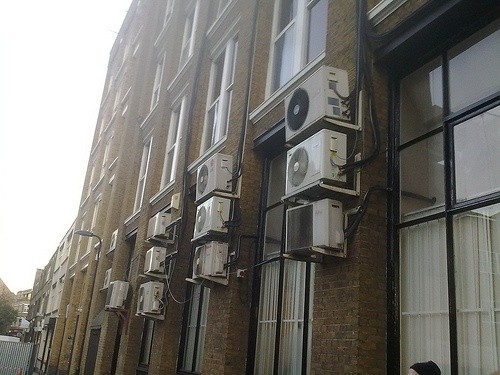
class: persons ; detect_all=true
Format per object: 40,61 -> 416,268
407,360 -> 441,375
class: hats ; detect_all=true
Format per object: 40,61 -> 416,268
409,360 -> 441,375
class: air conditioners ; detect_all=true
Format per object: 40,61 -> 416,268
99,268 -> 111,293
135,281 -> 164,314
144,247 -> 166,275
192,196 -> 231,239
195,153 -> 233,204
144,212 -> 171,241
192,241 -> 229,279
285,129 -> 348,196
284,65 -> 350,143
104,280 -> 130,312
284,198 -> 344,255
106,228 -> 118,257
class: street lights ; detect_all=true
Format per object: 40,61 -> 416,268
74,227 -> 103,375
